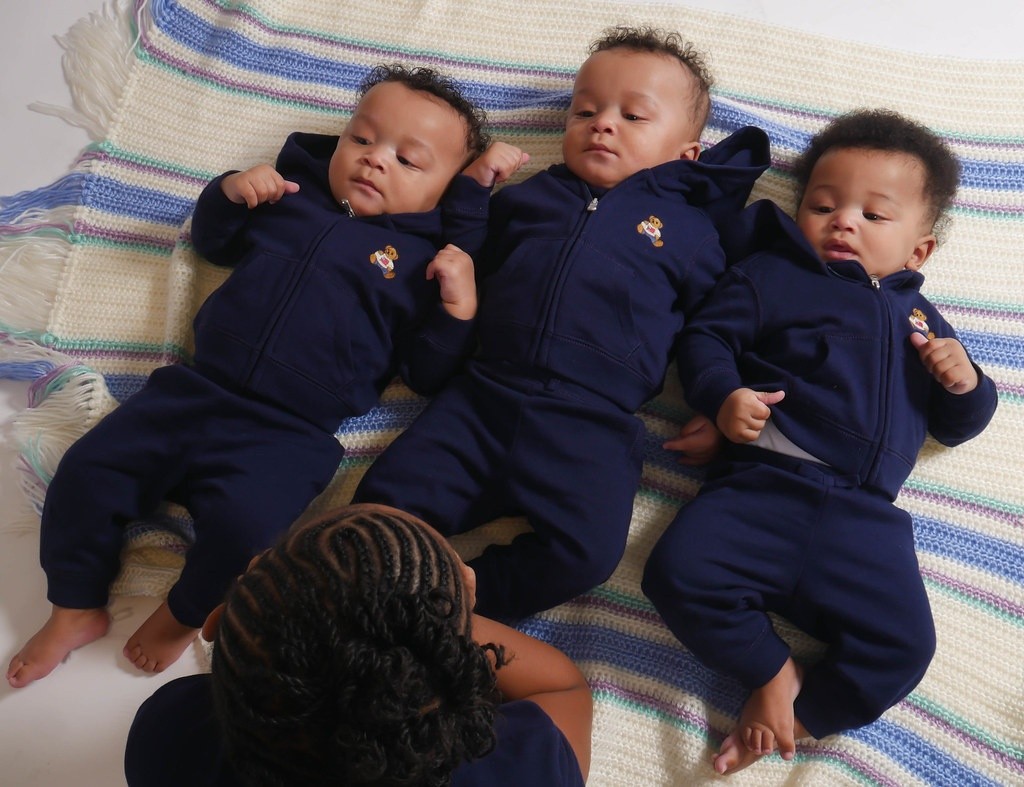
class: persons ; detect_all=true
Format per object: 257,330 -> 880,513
123,501 -> 594,787
4,69 -> 490,691
639,110 -> 999,776
347,31 -> 728,625
